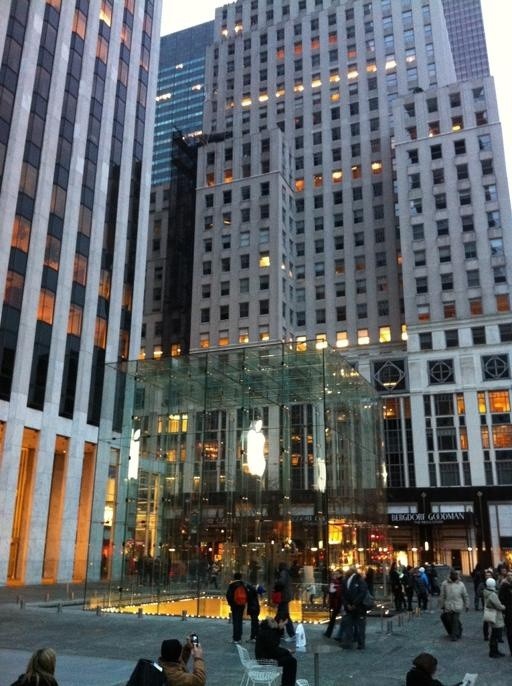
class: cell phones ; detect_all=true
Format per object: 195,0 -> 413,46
192,634 -> 198,647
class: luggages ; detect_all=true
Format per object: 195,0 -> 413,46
440,608 -> 462,635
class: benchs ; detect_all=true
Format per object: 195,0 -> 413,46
237,643 -> 284,685
301,602 -> 332,624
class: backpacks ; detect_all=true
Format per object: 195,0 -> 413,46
235,586 -> 247,605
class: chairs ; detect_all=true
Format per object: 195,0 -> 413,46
247,664 -> 283,686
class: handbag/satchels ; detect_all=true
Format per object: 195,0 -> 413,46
362,590 -> 374,608
483,608 -> 497,623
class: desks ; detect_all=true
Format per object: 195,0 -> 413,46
290,644 -> 343,686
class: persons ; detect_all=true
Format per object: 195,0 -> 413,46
11,647 -> 58,686
157,631 -> 205,685
226,559 -> 512,684
137,554 -> 171,586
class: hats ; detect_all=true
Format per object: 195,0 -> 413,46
161,640 -> 182,659
485,578 -> 496,590
